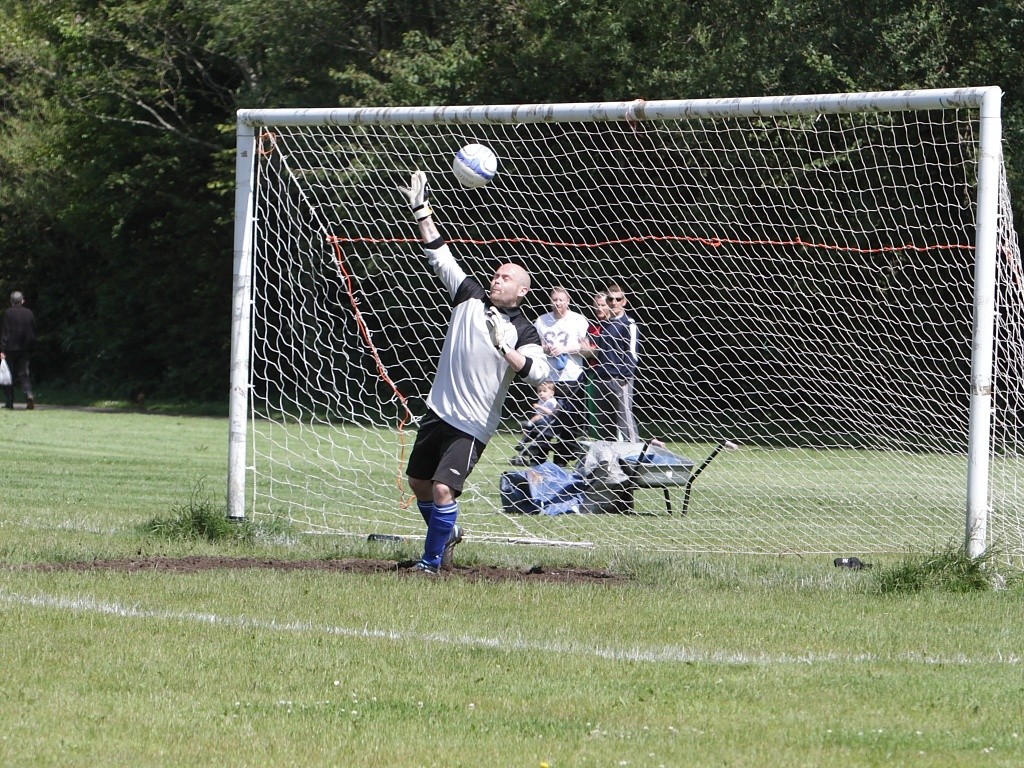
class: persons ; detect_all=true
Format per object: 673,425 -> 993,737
580,286 -> 639,467
528,382 -> 557,426
399,170 -> 549,574
530,286 -> 588,467
0,291 -> 36,409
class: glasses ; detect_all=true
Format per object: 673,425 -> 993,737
607,296 -> 623,302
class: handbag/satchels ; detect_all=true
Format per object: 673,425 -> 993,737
0,359 -> 12,385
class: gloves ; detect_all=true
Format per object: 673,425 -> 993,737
485,306 -> 511,356
398,171 -> 432,221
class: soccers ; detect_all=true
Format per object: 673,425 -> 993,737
451,143 -> 498,189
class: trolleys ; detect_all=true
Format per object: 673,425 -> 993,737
578,437 -> 738,519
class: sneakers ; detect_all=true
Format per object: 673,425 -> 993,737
441,525 -> 464,569
414,561 -> 438,575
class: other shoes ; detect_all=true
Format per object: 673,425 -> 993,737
556,462 -> 566,466
5,403 -> 13,409
26,394 -> 34,409
530,460 -> 539,466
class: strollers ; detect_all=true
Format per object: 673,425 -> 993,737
508,382 -> 588,467
